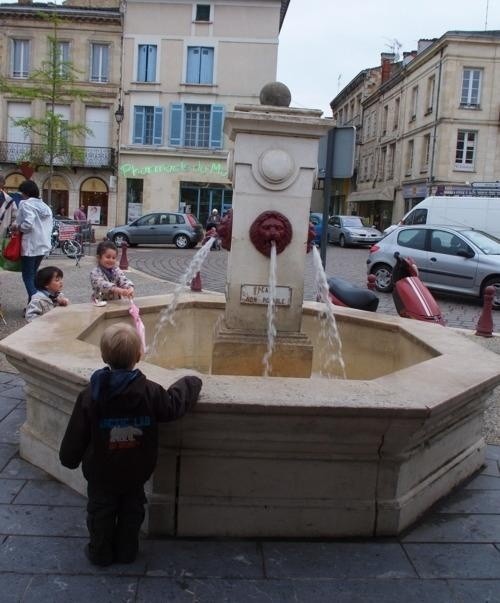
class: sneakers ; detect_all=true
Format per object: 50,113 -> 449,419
84,537 -> 139,568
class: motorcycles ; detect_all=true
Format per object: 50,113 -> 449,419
49,219 -> 92,258
317,251 -> 448,328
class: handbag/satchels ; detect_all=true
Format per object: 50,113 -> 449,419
0,225 -> 23,271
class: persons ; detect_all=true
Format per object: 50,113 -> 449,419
60,322 -> 202,566
205,226 -> 218,238
74,203 -> 87,220
90,239 -> 135,301
58,205 -> 65,215
206,208 -> 222,224
24,265 -> 70,323
3,178 -> 54,317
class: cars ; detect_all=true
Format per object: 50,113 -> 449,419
105,211 -> 204,249
51,215 -> 90,248
310,195 -> 500,311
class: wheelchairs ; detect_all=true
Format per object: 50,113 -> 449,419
203,225 -> 230,251
